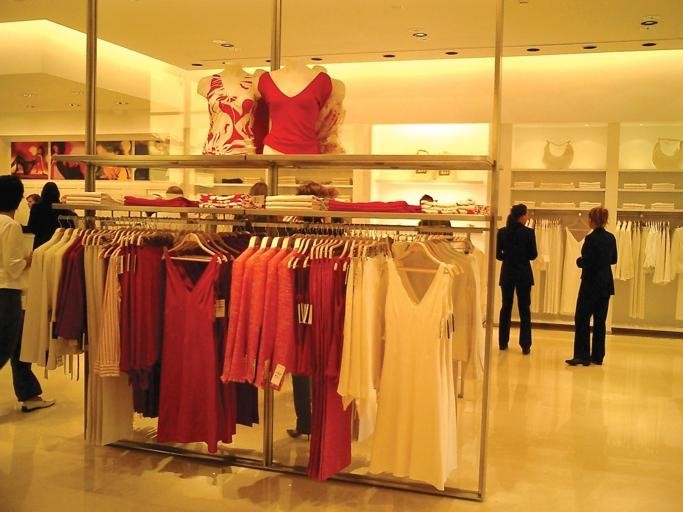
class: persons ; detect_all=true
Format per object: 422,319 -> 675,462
257,67 -> 332,153
146,186 -> 189,218
96,145 -> 121,180
196,68 -> 258,154
497,204 -> 538,355
50,145 -> 86,179
417,194 -> 455,236
565,207 -> 618,367
287,182 -> 339,441
10,145 -> 47,175
0,176 -> 78,412
232,182 -> 278,232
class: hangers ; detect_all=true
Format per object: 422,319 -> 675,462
33,216 -> 466,277
526,216 -> 674,232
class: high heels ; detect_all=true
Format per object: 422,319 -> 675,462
286,429 -> 311,441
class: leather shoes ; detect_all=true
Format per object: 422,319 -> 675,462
499,343 -> 508,350
21,399 -> 56,412
522,347 -> 530,354
565,357 -> 602,366
